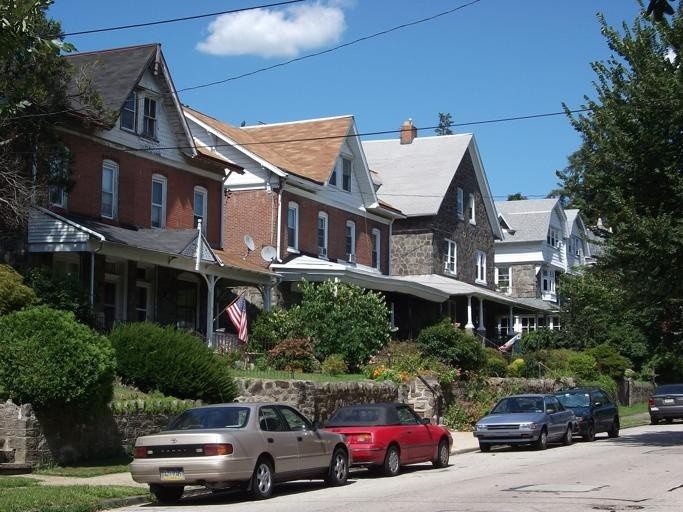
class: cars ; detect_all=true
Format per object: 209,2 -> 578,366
129,403 -> 355,507
648,384 -> 683,425
321,400 -> 454,478
473,386 -> 620,452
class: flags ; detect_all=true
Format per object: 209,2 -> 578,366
223,291 -> 249,343
